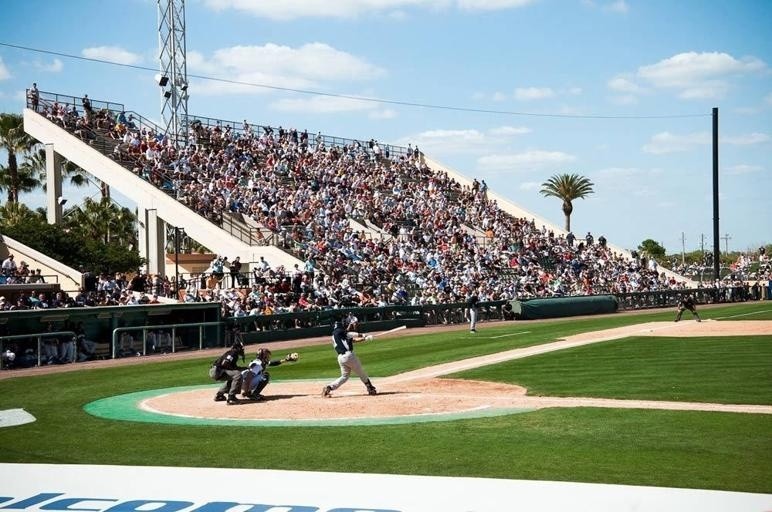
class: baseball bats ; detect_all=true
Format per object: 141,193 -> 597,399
373,325 -> 406,337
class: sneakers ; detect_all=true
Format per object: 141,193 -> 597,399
247,394 -> 264,400
213,395 -> 226,401
227,399 -> 240,405
369,389 -> 376,394
322,386 -> 330,397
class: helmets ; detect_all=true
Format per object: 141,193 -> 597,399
333,320 -> 341,329
256,348 -> 271,366
232,342 -> 245,356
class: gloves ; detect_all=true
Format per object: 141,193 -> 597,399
362,333 -> 373,342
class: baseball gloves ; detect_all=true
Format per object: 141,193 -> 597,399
285,353 -> 297,361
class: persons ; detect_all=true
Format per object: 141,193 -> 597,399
321,321 -> 376,395
29,82 -> 702,335
212,339 -> 299,404
667,245 -> 771,303
1,254 -> 171,367
